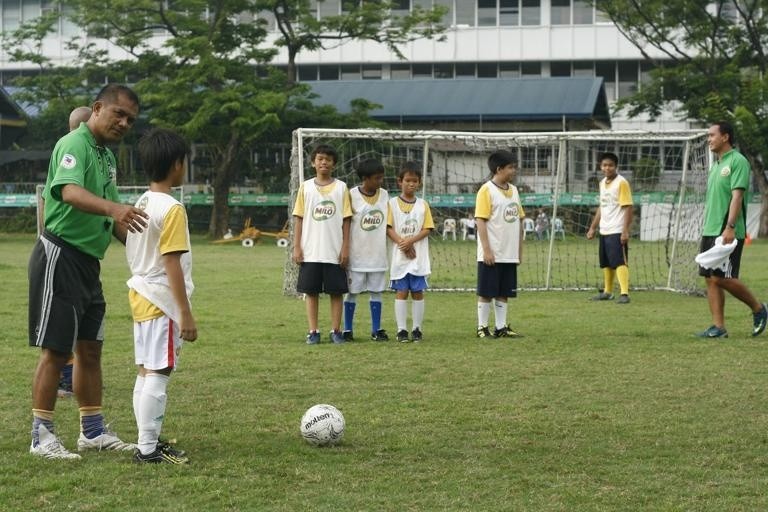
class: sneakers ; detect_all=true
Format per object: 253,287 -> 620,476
371,330 -> 388,341
77,433 -> 139,451
617,294 -> 630,305
28,436 -> 84,461
132,449 -> 191,466
157,441 -> 187,457
493,326 -> 524,337
698,326 -> 729,339
306,331 -> 321,345
396,330 -> 408,343
329,329 -> 353,343
751,303 -> 768,337
591,291 -> 614,302
476,327 -> 493,338
412,330 -> 422,342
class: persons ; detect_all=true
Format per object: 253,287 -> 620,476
342,157 -> 389,343
534,208 -> 549,241
39,106 -> 93,400
125,129 -> 199,466
26,83 -> 150,460
691,122 -> 768,340
473,149 -> 526,339
385,161 -> 436,343
585,152 -> 634,304
465,212 -> 477,240
291,143 -> 355,346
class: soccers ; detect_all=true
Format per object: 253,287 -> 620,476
300,404 -> 345,446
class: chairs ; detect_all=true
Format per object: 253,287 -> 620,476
442,219 -> 457,241
522,218 -> 534,240
460,218 -> 475,240
550,218 -> 565,239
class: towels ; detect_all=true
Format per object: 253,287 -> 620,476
694,237 -> 738,271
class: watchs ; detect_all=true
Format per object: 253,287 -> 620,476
727,223 -> 736,229
